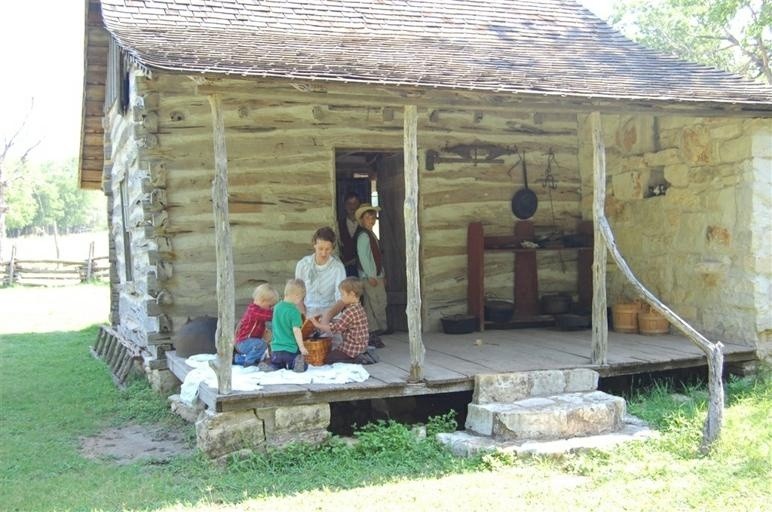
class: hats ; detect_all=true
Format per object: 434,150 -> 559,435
353,202 -> 383,227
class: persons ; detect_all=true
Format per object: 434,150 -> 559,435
339,193 -> 365,278
352,204 -> 390,347
270,276 -> 310,372
295,226 -> 351,346
311,276 -> 378,365
233,283 -> 281,369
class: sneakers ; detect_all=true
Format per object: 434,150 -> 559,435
376,329 -> 393,335
359,353 -> 375,363
367,349 -> 379,363
294,355 -> 304,372
369,331 -> 385,348
258,362 -> 274,372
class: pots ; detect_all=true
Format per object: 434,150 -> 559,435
174,314 -> 217,358
484,302 -> 516,321
439,313 -> 478,333
540,294 -> 570,315
512,162 -> 539,220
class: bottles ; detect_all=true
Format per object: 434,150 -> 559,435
647,187 -> 655,198
658,184 -> 665,195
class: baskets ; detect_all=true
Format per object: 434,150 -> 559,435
301,336 -> 333,367
